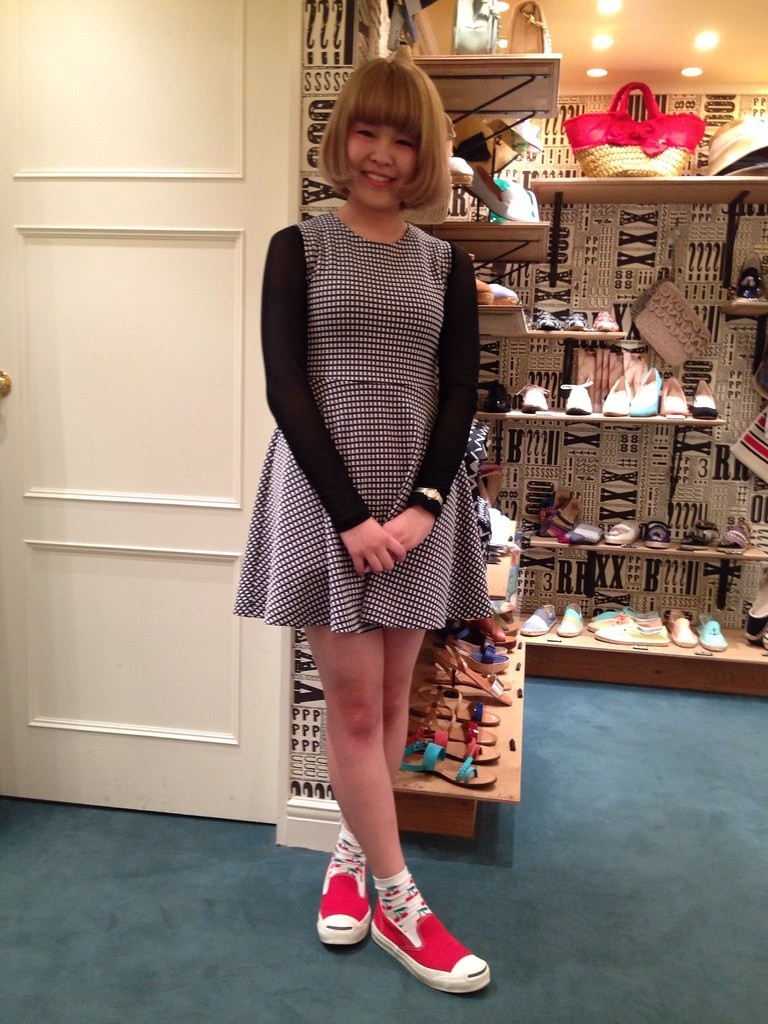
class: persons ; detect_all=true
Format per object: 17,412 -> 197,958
232,56 -> 495,993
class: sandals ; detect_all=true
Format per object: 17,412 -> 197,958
395,608 -> 519,789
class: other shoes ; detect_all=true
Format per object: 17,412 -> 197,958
464,164 -> 533,221
443,113 -> 473,185
469,263 -> 767,654
317,854 -> 371,945
370,895 -> 490,995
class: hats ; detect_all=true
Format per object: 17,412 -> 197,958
705,117 -> 768,178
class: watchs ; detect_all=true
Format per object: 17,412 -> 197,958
415,487 -> 443,506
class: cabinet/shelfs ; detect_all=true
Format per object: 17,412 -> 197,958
371,52 -> 768,837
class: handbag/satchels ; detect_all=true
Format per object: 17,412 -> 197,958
508,0 -> 551,54
562,81 -> 706,176
450,0 -> 501,54
